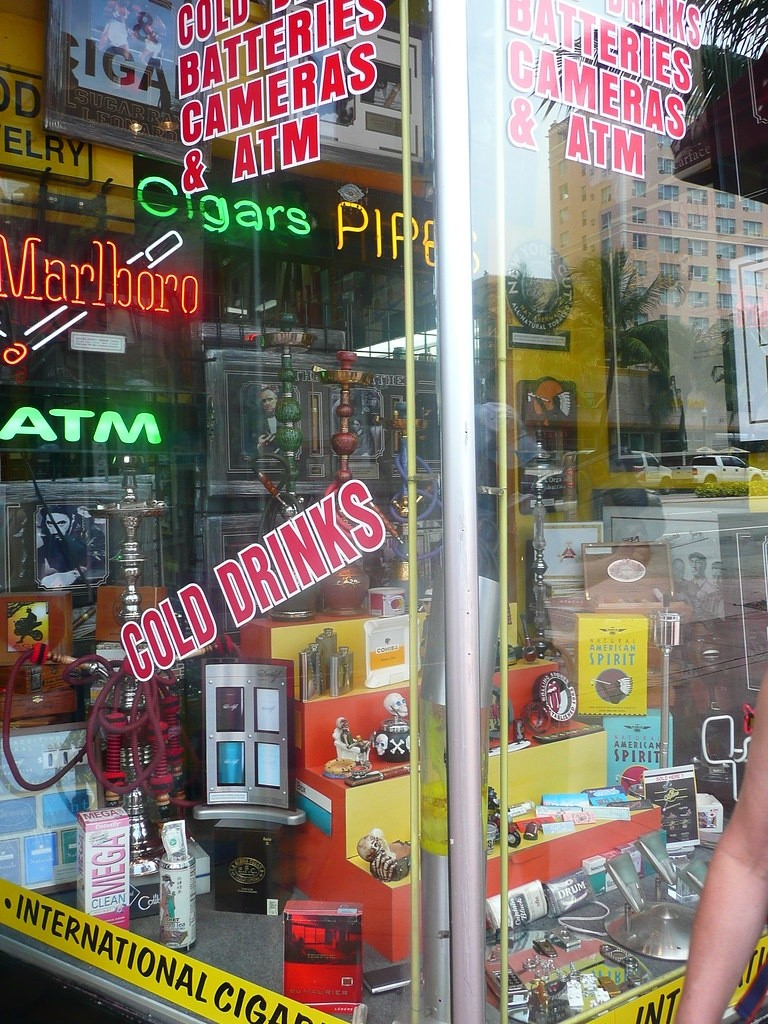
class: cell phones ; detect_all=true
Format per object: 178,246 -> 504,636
485,960 -> 528,998
361,963 -> 413,994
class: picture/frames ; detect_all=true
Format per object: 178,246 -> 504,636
44,0 -> 216,173
716,236 -> 767,452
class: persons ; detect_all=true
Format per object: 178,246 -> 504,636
478,403 -> 651,596
38,506 -> 105,587
671,670 -> 768,1024
672,552 -> 725,622
255,388 -> 283,453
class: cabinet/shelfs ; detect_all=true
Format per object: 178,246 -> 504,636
239,602 -> 661,963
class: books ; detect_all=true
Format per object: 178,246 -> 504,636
0,591 -> 73,695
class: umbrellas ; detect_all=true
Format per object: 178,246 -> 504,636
699,447 -> 750,453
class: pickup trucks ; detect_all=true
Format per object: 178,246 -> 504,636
669,454 -> 765,490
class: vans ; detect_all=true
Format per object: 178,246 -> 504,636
631,450 -> 673,495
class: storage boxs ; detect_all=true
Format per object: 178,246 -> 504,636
283,900 -> 363,1014
77,807 -> 129,929
0,587 -> 184,728
575,613 -> 673,786
582,829 -> 667,897
